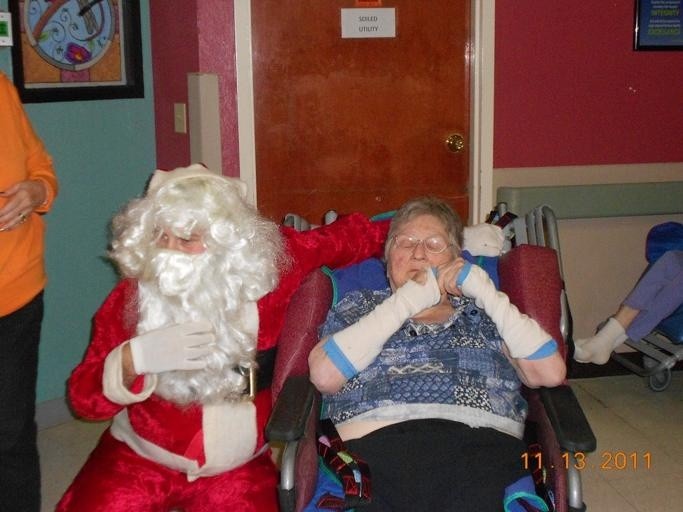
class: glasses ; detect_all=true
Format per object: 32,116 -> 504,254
393,232 -> 452,255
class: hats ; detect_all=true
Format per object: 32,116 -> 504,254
139,157 -> 250,210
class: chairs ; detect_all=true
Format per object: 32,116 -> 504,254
259,198 -> 596,511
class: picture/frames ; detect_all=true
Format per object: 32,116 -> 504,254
631,0 -> 682,53
6,0 -> 145,105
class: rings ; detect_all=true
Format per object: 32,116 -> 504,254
19,212 -> 27,222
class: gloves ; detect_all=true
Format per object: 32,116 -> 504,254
128,317 -> 218,374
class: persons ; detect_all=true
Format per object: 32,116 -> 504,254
0,71 -> 61,512
305,191 -> 571,512
51,161 -> 512,511
567,249 -> 683,366
